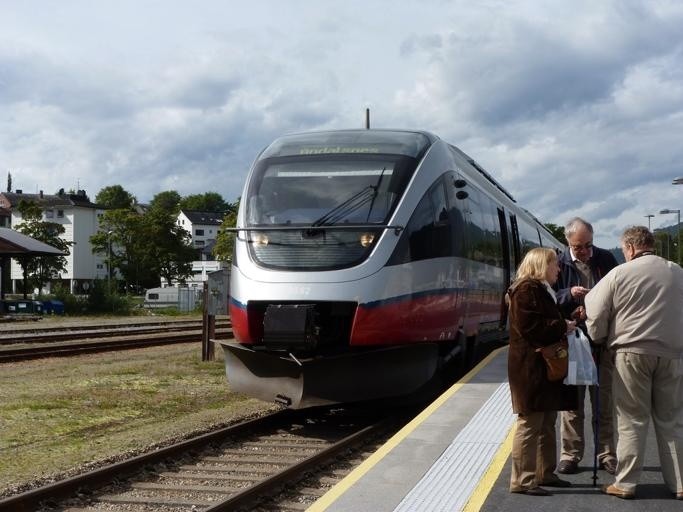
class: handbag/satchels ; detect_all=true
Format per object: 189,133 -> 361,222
563,318 -> 602,387
536,338 -> 569,382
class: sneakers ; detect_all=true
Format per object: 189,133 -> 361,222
542,473 -> 573,487
520,486 -> 553,496
558,459 -> 578,474
599,481 -> 634,499
603,459 -> 618,473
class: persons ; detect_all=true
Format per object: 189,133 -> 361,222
503,247 -> 584,495
553,216 -> 620,477
583,224 -> 683,501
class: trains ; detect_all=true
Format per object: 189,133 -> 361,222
206,108 -> 569,411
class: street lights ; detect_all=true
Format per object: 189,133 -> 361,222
659,209 -> 681,266
